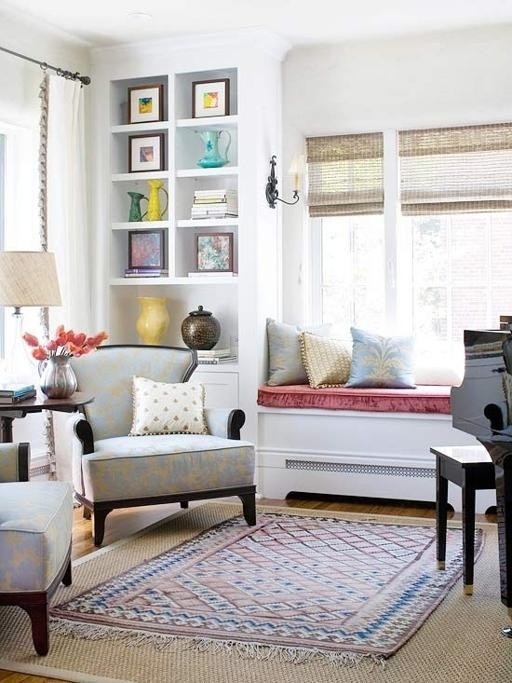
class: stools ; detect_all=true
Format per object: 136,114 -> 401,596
427,442 -> 501,597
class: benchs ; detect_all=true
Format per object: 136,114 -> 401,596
254,372 -> 497,515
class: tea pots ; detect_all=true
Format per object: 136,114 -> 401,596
195,129 -> 231,167
146,178 -> 169,220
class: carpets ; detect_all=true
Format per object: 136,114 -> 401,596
1,493 -> 512,683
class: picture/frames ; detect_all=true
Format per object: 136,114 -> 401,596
193,231 -> 235,273
126,228 -> 166,270
127,82 -> 166,125
128,132 -> 168,172
190,77 -> 231,119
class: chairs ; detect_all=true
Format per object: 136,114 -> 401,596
0,437 -> 78,657
53,337 -> 259,547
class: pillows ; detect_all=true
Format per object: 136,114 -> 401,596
264,310 -> 307,385
411,333 -> 468,386
297,331 -> 351,389
127,372 -> 211,438
342,324 -> 418,389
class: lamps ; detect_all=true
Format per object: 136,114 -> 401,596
264,147 -> 303,212
0,244 -> 63,326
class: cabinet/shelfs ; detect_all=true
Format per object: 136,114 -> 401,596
91,62 -> 284,494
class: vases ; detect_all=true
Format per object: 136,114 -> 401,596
34,355 -> 81,402
190,127 -> 235,169
143,178 -> 169,223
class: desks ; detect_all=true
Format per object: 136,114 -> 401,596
0,387 -> 98,443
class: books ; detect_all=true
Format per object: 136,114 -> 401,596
124,266 -> 168,274
0,389 -> 37,405
124,272 -> 168,278
189,189 -> 238,221
0,384 -> 38,395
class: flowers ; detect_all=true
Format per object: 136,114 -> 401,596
20,320 -> 111,358
